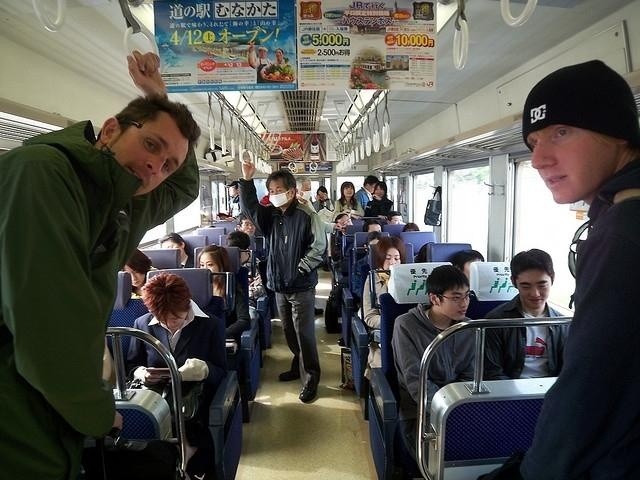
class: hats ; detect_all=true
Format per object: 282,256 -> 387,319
522,60 -> 640,153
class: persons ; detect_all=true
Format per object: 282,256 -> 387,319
361,236 -> 406,367
161,233 -> 194,268
354,175 -> 380,213
196,245 -> 251,340
402,223 -> 420,232
352,231 -> 384,298
223,182 -> 241,221
237,214 -> 257,238
364,181 -> 392,220
362,220 -> 382,234
334,181 -> 365,217
239,161 -> 328,402
482,248 -> 574,381
225,232 -> 265,317
330,214 -> 353,262
388,211 -> 405,225
312,186 -> 335,214
508,62 -> 640,480
391,266 -> 482,472
1,52 -> 201,478
123,248 -> 163,300
445,250 -> 484,284
126,273 -> 224,480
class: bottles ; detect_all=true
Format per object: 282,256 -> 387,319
311,132 -> 318,154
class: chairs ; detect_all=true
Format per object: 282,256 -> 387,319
210,221 -> 238,233
364,262 -> 455,479
426,243 -> 472,263
383,224 -> 406,236
219,234 -> 271,349
146,268 -> 244,480
400,231 -> 435,256
331,225 -> 364,270
335,232 -> 390,287
140,248 -> 181,269
193,246 -> 259,400
182,235 -> 208,254
349,242 -> 414,393
106,272 -> 146,384
197,228 -> 225,246
469,262 -> 520,320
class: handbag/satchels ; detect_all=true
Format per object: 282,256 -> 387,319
146,382 -> 203,422
325,291 -> 343,334
83,428 -> 191,480
424,186 -> 442,226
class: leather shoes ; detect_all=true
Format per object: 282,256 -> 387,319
279,370 -> 301,382
299,385 -> 317,403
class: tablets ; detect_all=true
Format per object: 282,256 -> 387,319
147,366 -> 171,375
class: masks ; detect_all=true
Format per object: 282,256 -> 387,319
268,190 -> 290,207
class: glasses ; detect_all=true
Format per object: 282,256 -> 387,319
437,292 -> 471,302
